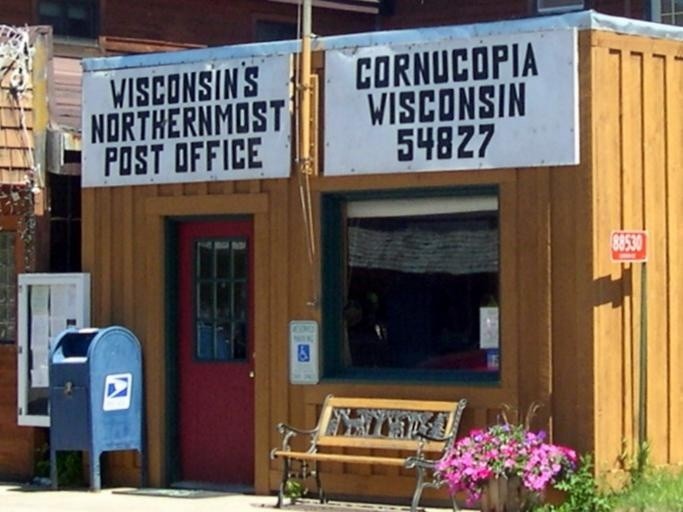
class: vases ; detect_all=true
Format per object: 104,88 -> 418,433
480,470 -> 534,511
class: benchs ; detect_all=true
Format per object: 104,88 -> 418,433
270,393 -> 468,512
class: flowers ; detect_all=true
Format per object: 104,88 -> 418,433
433,422 -> 577,509
